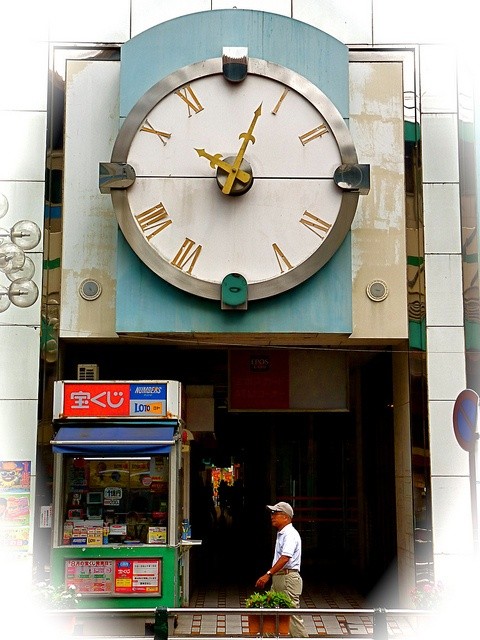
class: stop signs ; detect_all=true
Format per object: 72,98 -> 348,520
446,389 -> 480,457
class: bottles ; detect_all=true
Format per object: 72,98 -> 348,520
187,522 -> 191,538
181,519 -> 189,540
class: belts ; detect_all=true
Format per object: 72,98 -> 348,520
273,568 -> 297,575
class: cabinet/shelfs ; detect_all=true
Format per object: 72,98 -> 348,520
50,426 -> 194,609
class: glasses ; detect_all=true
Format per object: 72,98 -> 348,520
270,510 -> 283,514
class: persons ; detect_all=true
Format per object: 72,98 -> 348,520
255,501 -> 310,638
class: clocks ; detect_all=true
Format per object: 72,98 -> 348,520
100,47 -> 370,311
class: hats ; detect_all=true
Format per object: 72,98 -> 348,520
265,501 -> 294,519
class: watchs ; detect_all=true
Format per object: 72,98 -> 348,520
266,570 -> 272,577
